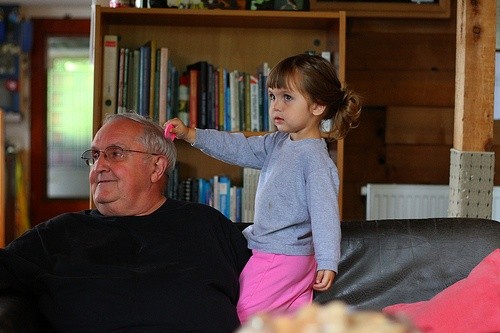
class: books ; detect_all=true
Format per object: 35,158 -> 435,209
116,0 -> 309,12
102,35 -> 332,223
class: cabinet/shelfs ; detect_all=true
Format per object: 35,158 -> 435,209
90,5 -> 346,223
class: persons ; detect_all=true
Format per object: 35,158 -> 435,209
237,248 -> 500,333
0,109 -> 253,333
164,54 -> 364,327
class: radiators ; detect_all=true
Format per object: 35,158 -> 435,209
362,184 -> 500,221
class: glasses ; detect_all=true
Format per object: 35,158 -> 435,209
80,146 -> 158,166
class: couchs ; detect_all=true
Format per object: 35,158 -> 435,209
313,216 -> 500,333
